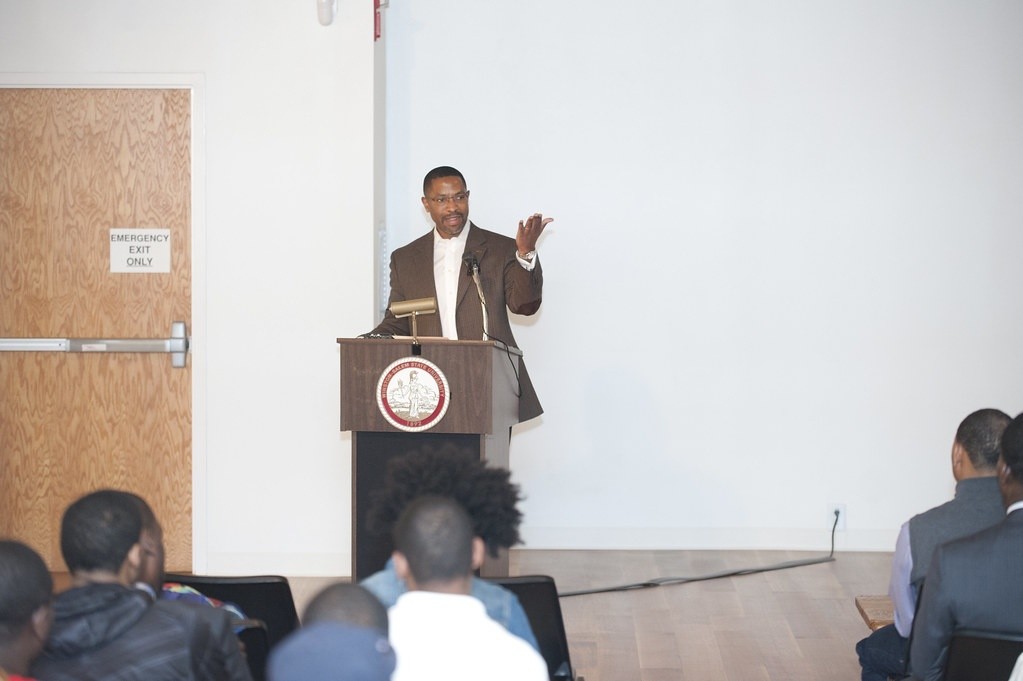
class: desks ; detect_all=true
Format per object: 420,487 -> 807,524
855,595 -> 894,631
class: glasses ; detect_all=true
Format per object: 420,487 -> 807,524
424,192 -> 468,206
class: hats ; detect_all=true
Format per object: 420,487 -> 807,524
264,620 -> 397,681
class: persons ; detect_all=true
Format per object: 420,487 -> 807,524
0,489 -> 267,681
857,407 -> 1023,681
267,442 -> 551,681
355,167 -> 553,442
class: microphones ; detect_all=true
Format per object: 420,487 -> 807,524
462,250 -> 480,276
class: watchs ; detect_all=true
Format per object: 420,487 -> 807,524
520,250 -> 535,260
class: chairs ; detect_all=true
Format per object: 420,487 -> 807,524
481,573 -> 574,681
944,628 -> 1023,681
164,572 -> 300,634
